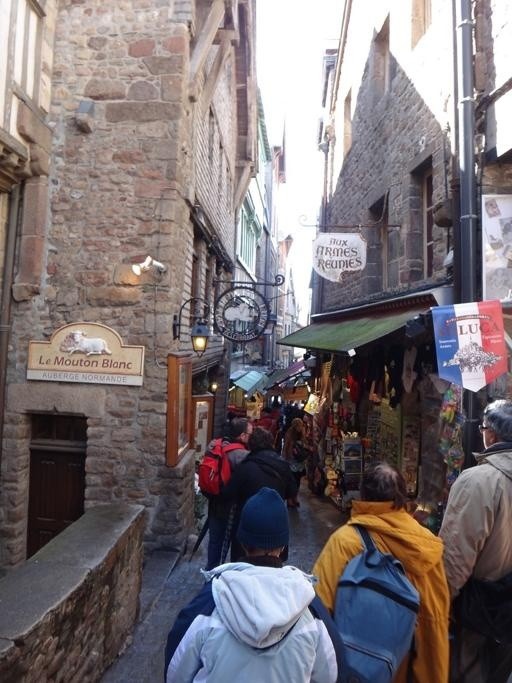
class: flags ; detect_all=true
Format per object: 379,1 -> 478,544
432,299 -> 508,392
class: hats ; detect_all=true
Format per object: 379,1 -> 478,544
235,487 -> 290,551
247,427 -> 274,450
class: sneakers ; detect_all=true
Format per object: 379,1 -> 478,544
287,501 -> 300,508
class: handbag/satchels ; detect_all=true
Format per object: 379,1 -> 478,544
293,439 -> 313,461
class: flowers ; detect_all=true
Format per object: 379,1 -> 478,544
344,432 -> 361,440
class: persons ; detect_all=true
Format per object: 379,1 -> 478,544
308,459 -> 452,683
161,485 -> 351,683
437,395 -> 512,681
198,408 -> 312,574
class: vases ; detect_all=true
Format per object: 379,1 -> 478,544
345,439 -> 360,445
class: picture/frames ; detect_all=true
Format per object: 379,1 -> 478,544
168,352 -> 193,468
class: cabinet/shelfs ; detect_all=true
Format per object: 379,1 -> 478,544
330,444 -> 362,512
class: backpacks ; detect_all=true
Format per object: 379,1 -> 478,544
198,437 -> 244,496
334,522 -> 422,680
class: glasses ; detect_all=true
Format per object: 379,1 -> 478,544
477,423 -> 492,433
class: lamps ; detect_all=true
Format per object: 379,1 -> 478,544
173,297 -> 211,357
